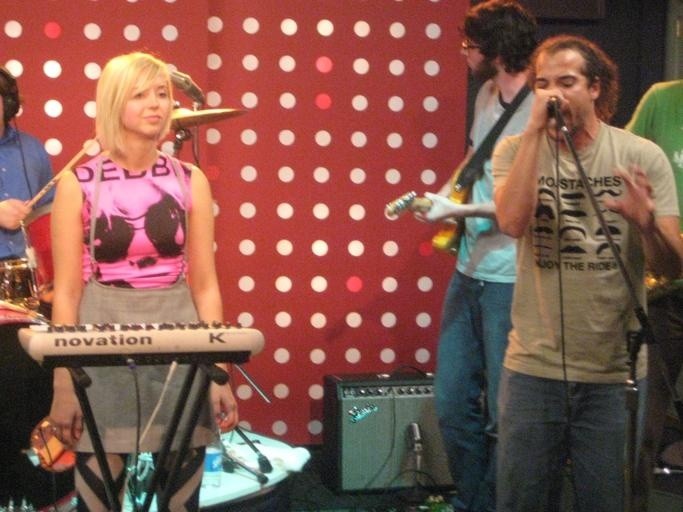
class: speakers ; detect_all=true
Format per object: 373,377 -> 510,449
324,371 -> 458,493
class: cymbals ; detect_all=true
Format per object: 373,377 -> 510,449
170,105 -> 244,126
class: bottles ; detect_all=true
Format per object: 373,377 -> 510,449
198,419 -> 224,487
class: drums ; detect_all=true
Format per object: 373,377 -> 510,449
0,257 -> 34,311
20,204 -> 53,291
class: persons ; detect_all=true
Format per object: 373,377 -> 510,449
410,0 -> 544,511
46,49 -> 239,512
621,76 -> 682,512
489,32 -> 682,511
0,68 -> 57,262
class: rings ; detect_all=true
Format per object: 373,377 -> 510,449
226,402 -> 237,413
51,427 -> 57,432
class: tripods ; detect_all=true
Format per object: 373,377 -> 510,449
189,126 -> 267,484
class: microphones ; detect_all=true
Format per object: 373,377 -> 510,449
165,64 -> 191,89
548,97 -> 559,117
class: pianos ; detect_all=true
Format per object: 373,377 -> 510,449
17,319 -> 265,367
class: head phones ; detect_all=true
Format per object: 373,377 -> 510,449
0,68 -> 19,115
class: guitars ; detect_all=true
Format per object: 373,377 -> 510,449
384,171 -> 470,254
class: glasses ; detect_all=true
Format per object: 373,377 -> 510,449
462,40 -> 480,48
